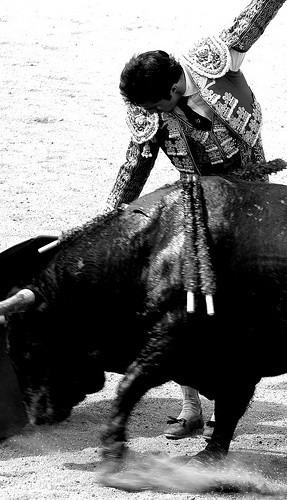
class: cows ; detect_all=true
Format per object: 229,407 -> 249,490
0,175 -> 287,470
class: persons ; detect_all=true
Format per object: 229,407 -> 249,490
103,0 -> 287,442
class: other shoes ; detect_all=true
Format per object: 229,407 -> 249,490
163,414 -> 204,439
202,420 -> 215,440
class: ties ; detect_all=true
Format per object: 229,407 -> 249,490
177,96 -> 212,131
